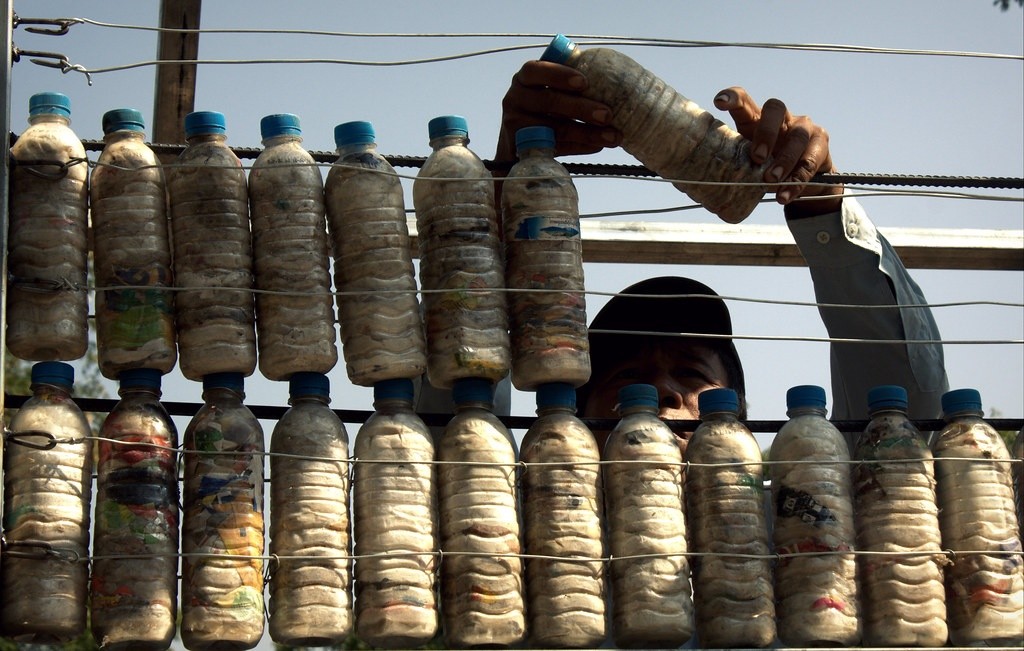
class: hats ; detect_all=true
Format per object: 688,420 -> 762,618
576,277 -> 746,396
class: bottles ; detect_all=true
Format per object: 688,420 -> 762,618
539,33 -> 782,224
0,92 -> 1024,650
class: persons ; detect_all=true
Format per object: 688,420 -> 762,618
413,60 -> 951,459
